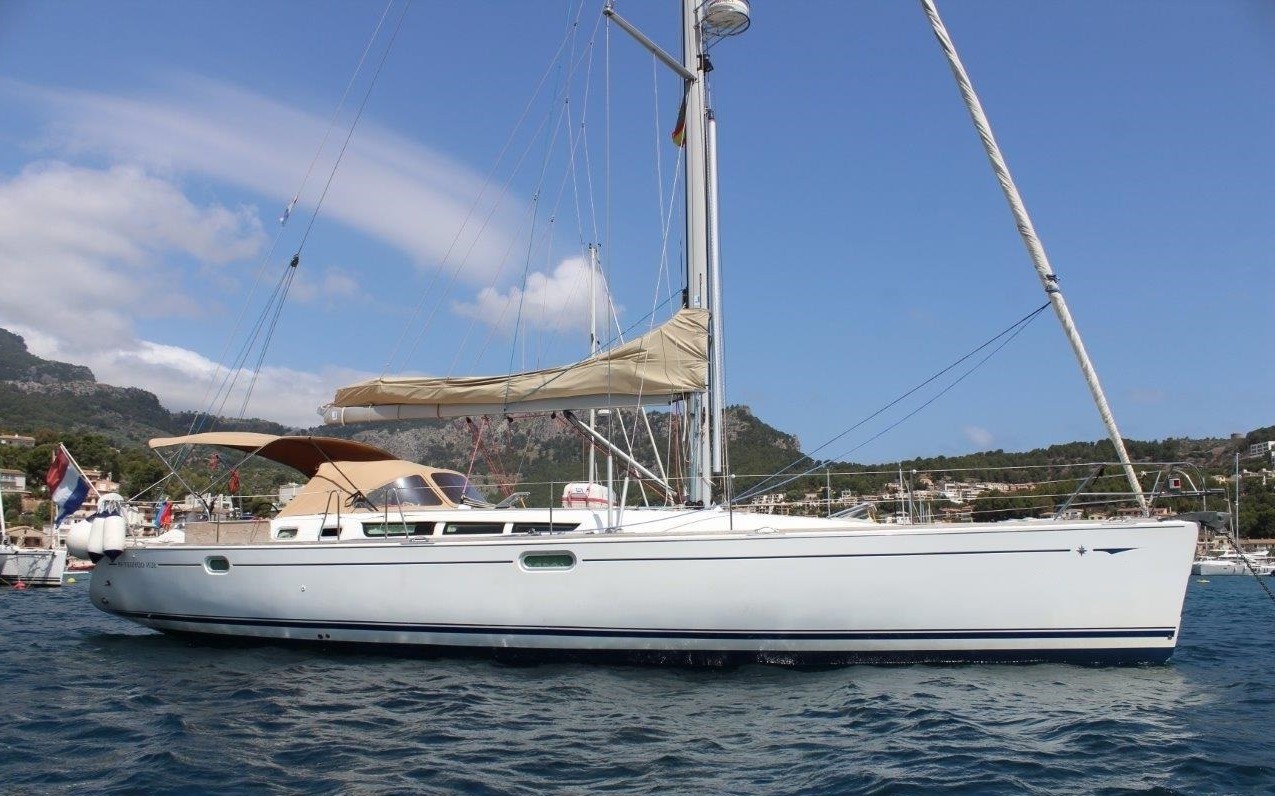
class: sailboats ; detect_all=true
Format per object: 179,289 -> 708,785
0,0 -> 1275,658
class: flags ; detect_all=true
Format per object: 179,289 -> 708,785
45,447 -> 92,529
